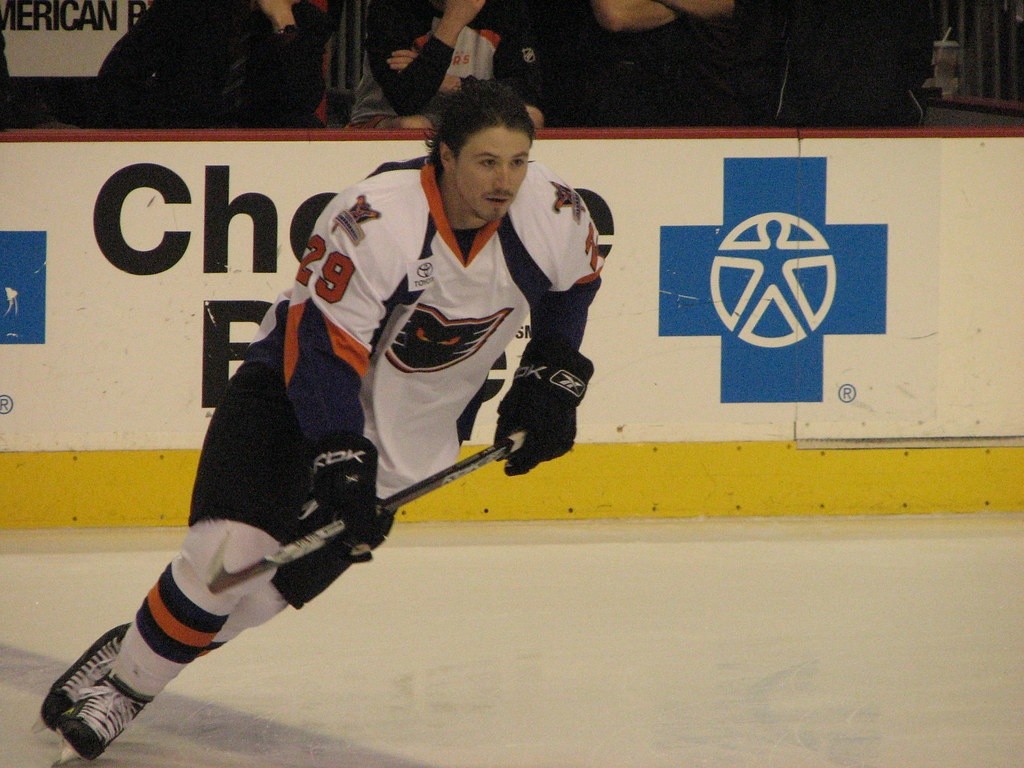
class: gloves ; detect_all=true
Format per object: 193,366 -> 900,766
493,339 -> 595,477
296,431 -> 398,562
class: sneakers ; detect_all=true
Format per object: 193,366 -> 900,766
31,621 -> 134,733
50,668 -> 157,768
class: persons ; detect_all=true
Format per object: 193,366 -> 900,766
64,0 -> 933,130
38,78 -> 606,762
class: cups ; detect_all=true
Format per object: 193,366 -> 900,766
935,41 -> 960,95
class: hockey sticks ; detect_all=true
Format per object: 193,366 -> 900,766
201,428 -> 532,597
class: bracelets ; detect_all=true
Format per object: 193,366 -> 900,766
273,24 -> 296,35
